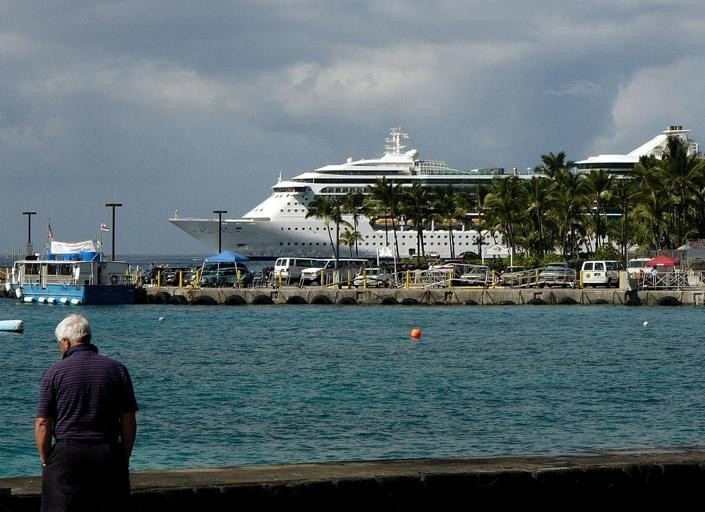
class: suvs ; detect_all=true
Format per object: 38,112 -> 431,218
540,261 -> 576,288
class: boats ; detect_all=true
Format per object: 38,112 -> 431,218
4,220 -> 139,306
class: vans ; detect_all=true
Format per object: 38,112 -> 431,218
300,257 -> 371,285
274,256 -> 318,284
581,260 -> 625,287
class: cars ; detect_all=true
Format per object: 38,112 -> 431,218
460,264 -> 491,285
627,256 -> 656,278
500,264 -> 528,283
149,262 -> 251,287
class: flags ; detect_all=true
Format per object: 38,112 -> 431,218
47,225 -> 53,242
101,224 -> 109,232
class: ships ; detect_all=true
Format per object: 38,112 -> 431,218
168,126 -> 699,273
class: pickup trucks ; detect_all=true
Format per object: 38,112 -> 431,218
354,268 -> 389,287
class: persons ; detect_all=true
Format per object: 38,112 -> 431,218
35,315 -> 138,512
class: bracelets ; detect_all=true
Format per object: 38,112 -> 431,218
40,457 -> 47,464
